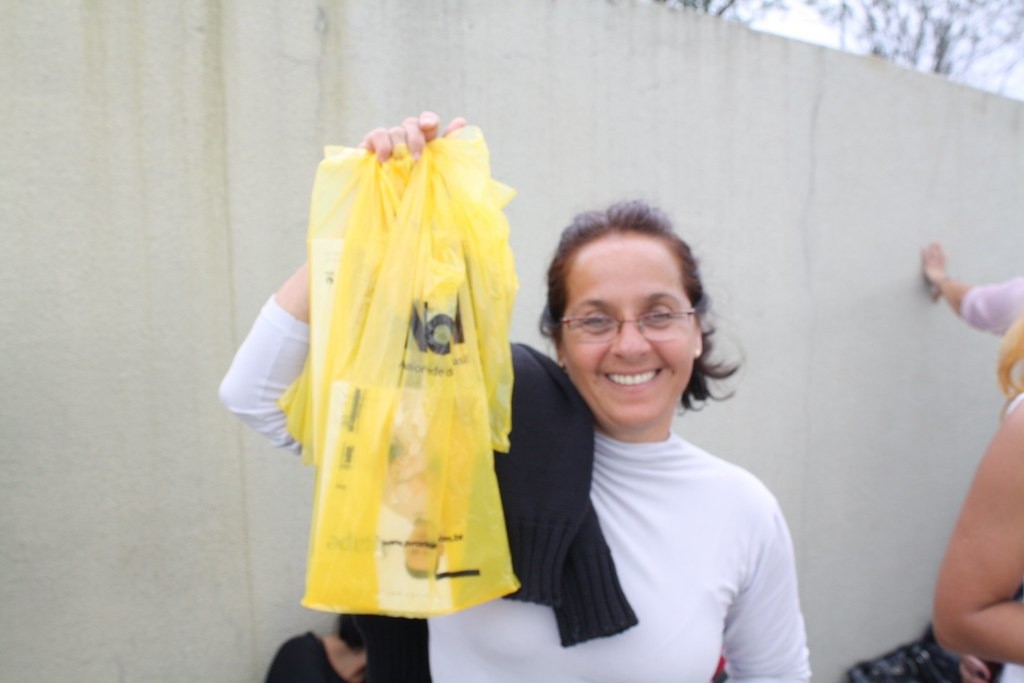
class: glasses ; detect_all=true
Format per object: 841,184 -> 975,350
559,309 -> 696,343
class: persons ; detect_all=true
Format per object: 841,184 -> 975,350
265,612 -> 367,683
217,109 -> 815,683
923,243 -> 1024,683
